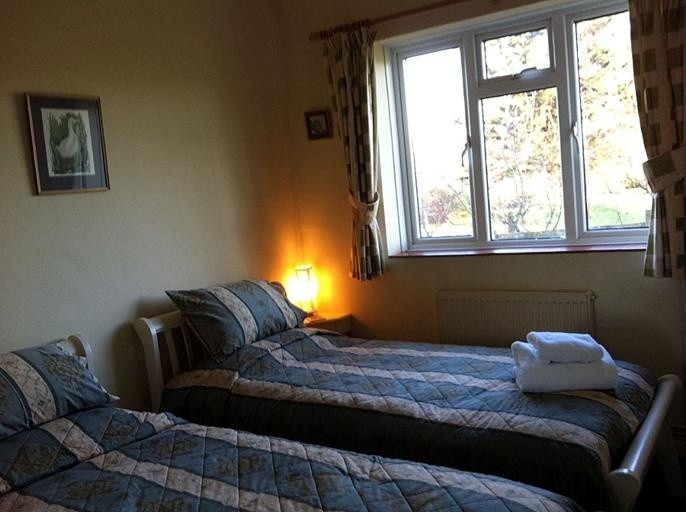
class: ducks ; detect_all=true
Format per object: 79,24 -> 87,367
55,117 -> 79,159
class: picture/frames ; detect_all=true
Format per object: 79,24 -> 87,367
25,88 -> 110,197
304,109 -> 333,140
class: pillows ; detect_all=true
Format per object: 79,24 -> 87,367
166,267 -> 310,364
